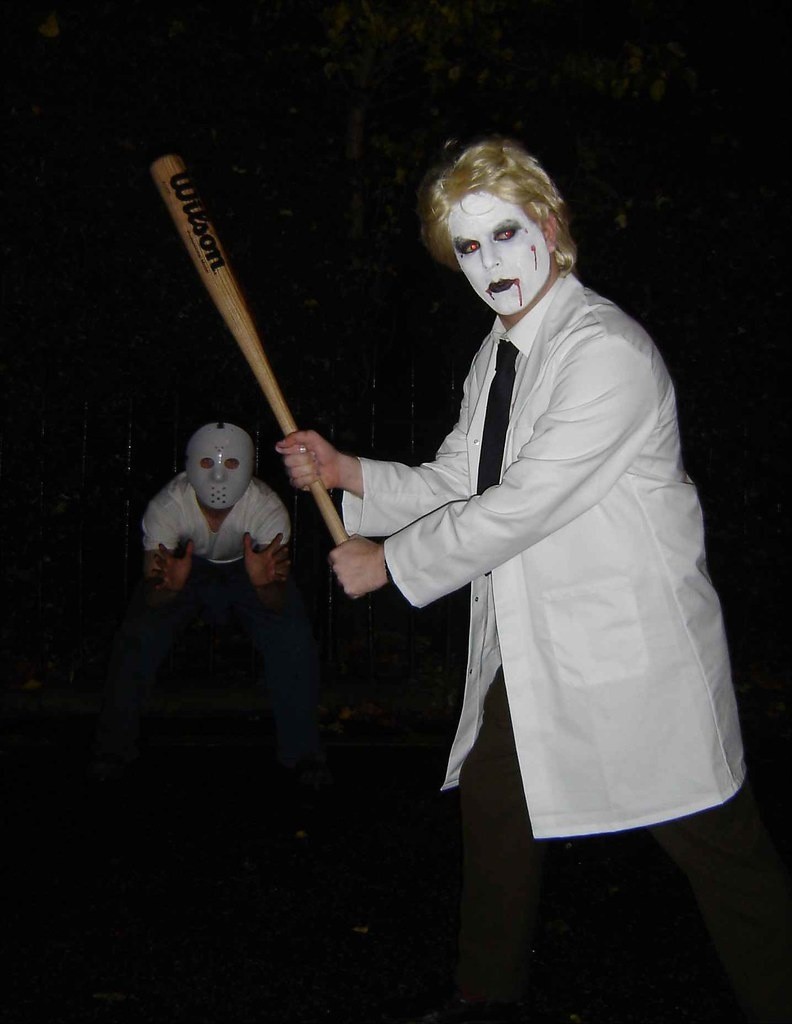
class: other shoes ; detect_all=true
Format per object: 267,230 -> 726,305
86,755 -> 124,782
421,987 -> 520,1024
284,761 -> 333,785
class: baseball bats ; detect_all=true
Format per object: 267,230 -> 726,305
151,154 -> 350,546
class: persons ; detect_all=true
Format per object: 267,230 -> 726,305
85,420 -> 329,816
275,142 -> 792,1024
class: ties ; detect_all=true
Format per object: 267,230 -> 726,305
476,339 -> 519,576
